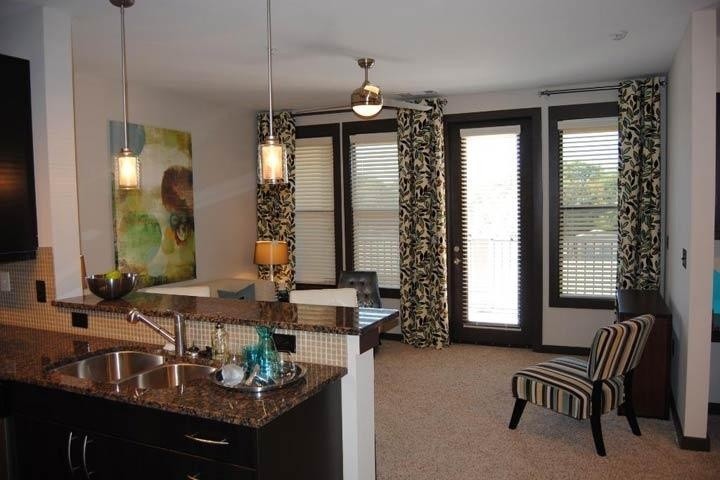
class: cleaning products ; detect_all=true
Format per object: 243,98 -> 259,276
210,322 -> 230,361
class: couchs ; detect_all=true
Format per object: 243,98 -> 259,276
137,276 -> 278,303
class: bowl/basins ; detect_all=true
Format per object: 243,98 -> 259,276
87,272 -> 138,299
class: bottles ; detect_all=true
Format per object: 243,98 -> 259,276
211,319 -> 228,361
254,322 -> 280,380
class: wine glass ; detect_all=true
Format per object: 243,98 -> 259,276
230,343 -> 238,361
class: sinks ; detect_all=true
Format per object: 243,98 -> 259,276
42,345 -> 166,385
117,363 -> 220,390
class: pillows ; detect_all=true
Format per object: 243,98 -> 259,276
216,282 -> 256,301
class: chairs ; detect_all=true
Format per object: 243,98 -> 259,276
287,286 -> 359,308
506,312 -> 661,456
338,269 -> 386,359
146,284 -> 210,299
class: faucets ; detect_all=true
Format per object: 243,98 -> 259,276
127,306 -> 188,355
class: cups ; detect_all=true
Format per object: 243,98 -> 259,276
244,346 -> 295,383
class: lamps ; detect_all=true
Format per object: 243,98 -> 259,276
348,99 -> 383,120
254,1 -> 292,189
252,238 -> 290,284
108,1 -> 144,197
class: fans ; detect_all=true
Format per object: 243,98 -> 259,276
289,56 -> 434,116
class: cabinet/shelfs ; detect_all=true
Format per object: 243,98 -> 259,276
0,50 -> 41,266
1,375 -> 150,480
608,287 -> 675,424
146,377 -> 344,480
